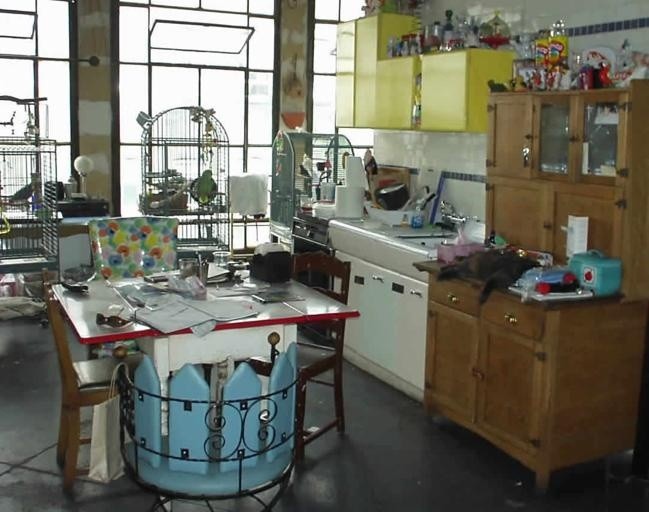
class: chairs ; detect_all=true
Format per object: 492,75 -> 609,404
41,216 -> 352,512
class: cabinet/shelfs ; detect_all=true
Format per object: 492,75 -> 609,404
378,50 -> 518,133
335,13 -> 421,128
412,76 -> 649,495
334,250 -> 429,402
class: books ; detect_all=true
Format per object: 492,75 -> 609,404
132,300 -> 260,334
506,284 -> 594,304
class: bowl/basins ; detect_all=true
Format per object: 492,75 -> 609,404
364,200 -> 428,225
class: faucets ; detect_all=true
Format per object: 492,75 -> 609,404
439,200 -> 467,224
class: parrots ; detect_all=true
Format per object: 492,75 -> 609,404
196,170 -> 214,205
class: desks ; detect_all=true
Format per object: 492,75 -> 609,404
54,269 -> 360,437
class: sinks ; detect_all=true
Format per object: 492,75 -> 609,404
395,231 -> 456,249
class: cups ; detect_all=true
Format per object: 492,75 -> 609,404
213,252 -> 231,271
179,257 -> 198,279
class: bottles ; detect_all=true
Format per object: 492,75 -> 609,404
400,8 -> 454,56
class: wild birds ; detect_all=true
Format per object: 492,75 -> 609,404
9,183 -> 34,203
